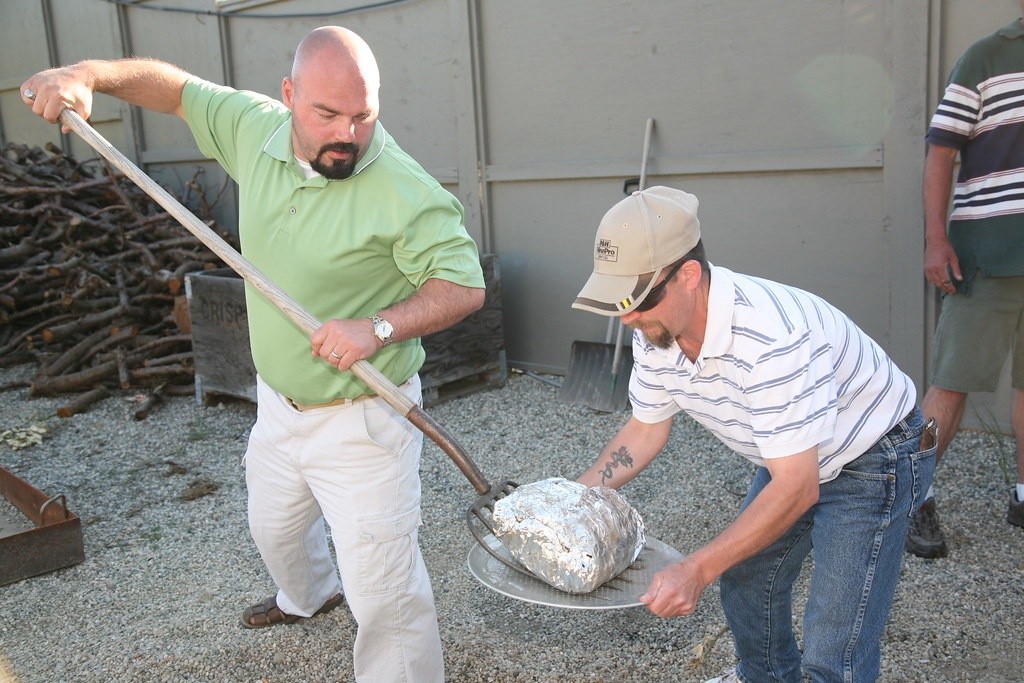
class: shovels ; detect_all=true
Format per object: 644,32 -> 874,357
557,179 -> 639,414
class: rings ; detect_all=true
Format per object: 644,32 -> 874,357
331,350 -> 340,359
24,87 -> 36,101
942,279 -> 953,287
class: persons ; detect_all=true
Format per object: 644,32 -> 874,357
570,184 -> 940,683
904,0 -> 1024,560
20,24 -> 484,683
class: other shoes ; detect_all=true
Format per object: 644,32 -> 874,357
705,668 -> 742,683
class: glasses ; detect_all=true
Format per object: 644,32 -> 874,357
634,257 -> 703,311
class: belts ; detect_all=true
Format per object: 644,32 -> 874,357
286,380 -> 407,413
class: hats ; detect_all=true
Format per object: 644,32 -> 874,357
572,186 -> 700,316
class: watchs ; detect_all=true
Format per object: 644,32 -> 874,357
366,313 -> 393,348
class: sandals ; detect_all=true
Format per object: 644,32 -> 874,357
240,593 -> 343,629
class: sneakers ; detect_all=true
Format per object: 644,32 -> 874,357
1007,490 -> 1024,527
904,497 -> 947,557
